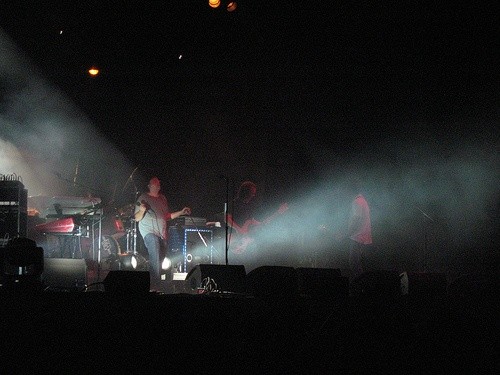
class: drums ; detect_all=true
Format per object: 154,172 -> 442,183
102,215 -> 126,240
89,235 -> 120,263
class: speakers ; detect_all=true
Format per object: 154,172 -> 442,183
185,264 -> 450,299
103,271 -> 151,295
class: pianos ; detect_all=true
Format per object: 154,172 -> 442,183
34,217 -> 86,236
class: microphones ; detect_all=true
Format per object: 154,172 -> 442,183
141,200 -> 155,213
219,174 -> 228,180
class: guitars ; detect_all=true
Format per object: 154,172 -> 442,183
227,202 -> 289,254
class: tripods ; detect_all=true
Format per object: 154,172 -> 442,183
80,208 -> 103,293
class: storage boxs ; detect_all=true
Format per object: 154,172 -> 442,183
0,180 -> 27,247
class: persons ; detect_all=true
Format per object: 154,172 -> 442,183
334,176 -> 374,272
225,181 -> 262,265
132,176 -> 192,294
